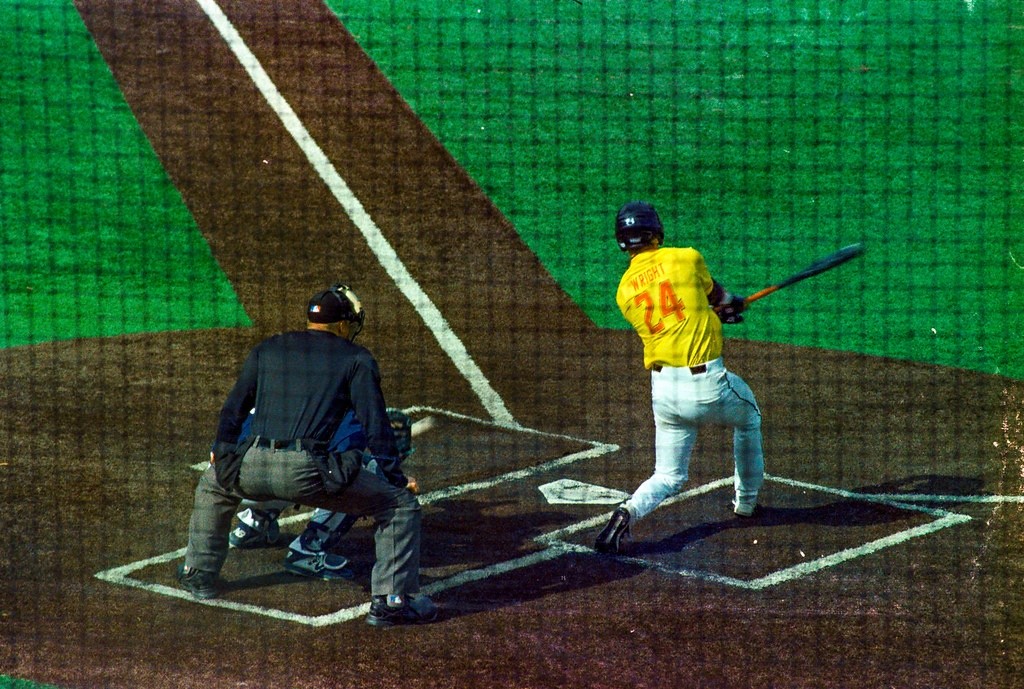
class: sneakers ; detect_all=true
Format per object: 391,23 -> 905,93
593,502 -> 637,553
175,562 -> 220,599
733,499 -> 756,517
227,507 -> 281,550
363,592 -> 438,627
282,520 -> 351,580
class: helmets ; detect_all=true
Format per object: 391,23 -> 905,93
613,202 -> 665,251
386,407 -> 412,452
307,280 -> 366,323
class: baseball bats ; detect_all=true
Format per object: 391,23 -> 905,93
716,239 -> 868,310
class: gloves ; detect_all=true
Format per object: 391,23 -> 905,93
720,294 -> 748,323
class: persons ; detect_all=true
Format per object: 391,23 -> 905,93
176,283 -> 426,628
595,202 -> 765,558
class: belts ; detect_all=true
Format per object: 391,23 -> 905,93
253,436 -> 291,450
651,364 -> 705,375
